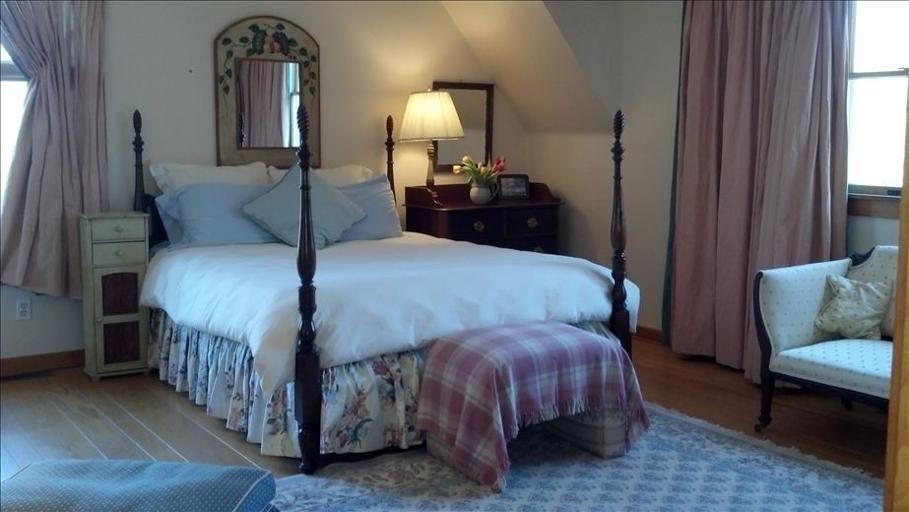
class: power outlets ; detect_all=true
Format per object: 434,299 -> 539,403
16,298 -> 31,320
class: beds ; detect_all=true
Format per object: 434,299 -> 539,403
132,101 -> 641,475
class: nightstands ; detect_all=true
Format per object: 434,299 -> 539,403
402,183 -> 565,255
80,212 -> 151,382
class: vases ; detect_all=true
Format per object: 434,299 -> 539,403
469,183 -> 499,205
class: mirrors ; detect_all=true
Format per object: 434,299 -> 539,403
433,82 -> 494,172
214,15 -> 321,170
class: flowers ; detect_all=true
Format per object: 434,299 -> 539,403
453,153 -> 508,185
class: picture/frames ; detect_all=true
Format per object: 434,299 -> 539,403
498,174 -> 529,201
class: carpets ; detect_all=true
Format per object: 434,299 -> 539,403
271,396 -> 885,512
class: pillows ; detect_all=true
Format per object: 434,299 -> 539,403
155,183 -> 280,250
268,165 -> 374,184
240,163 -> 367,250
149,160 -> 268,193
336,174 -> 402,241
814,274 -> 893,340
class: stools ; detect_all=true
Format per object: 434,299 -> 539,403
0,459 -> 280,512
416,321 -> 650,494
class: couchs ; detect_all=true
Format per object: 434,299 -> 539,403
753,244 -> 899,427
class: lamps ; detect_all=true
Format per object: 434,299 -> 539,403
397,88 -> 465,208
